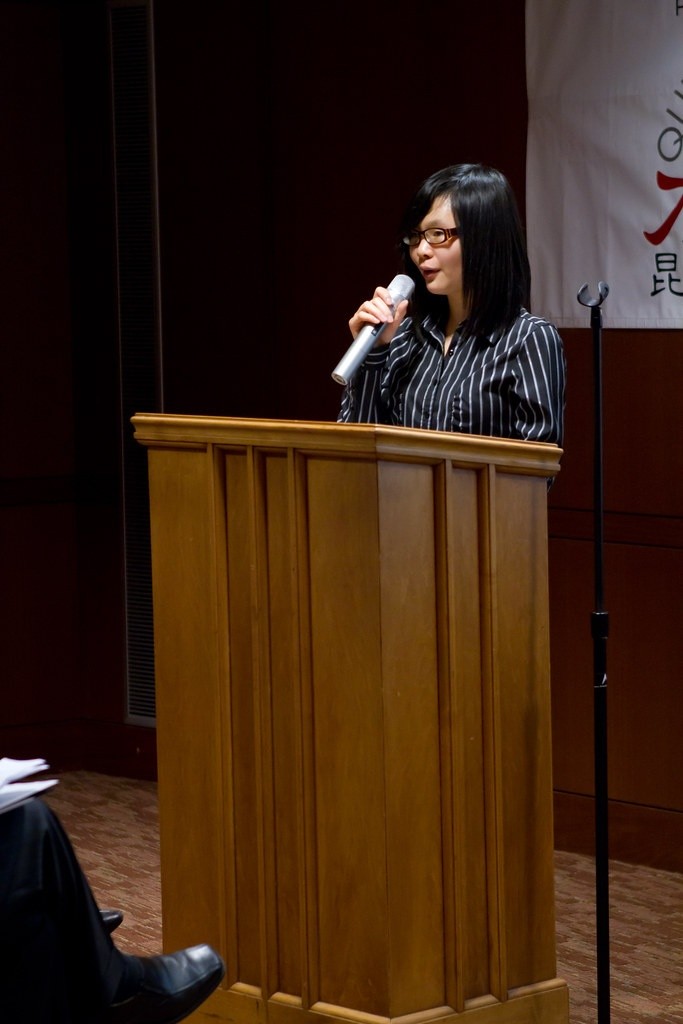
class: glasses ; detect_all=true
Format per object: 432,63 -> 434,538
402,227 -> 461,246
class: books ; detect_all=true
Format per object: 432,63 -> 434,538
0,755 -> 59,816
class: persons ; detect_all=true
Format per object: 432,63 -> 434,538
337,163 -> 566,493
0,797 -> 226,1024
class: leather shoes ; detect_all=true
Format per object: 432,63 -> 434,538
105,944 -> 224,1024
100,910 -> 122,933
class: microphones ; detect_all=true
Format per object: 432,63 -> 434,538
332,274 -> 414,386
